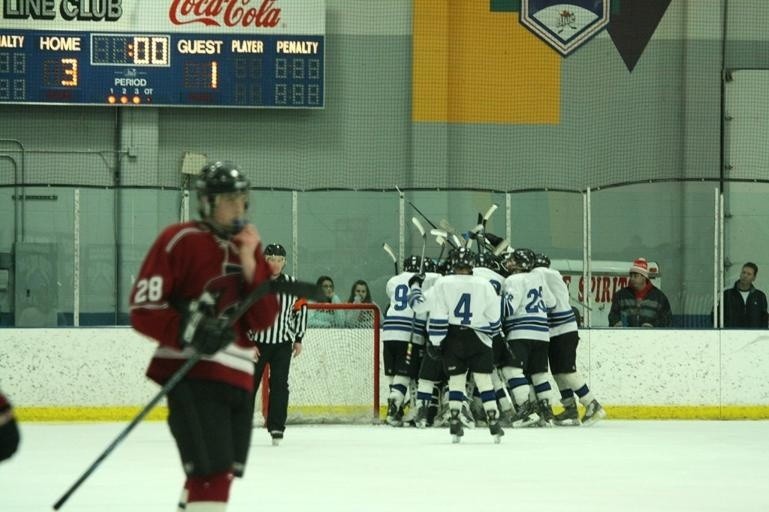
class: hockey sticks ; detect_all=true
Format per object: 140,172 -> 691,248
52,280 -> 328,510
382,186 -> 592,374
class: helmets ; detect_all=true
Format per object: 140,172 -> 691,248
263,244 -> 286,266
404,247 -> 550,275
195,160 -> 250,199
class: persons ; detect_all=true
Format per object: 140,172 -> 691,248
346,280 -> 385,329
381,248 -> 605,427
127,161 -> 282,510
306,275 -> 345,328
429,263 -> 505,437
608,255 -> 673,329
711,260 -> 767,329
254,236 -> 309,448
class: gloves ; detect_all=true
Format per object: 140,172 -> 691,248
178,312 -> 237,354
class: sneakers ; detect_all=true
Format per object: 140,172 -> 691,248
487,419 -> 504,434
387,402 -> 603,426
271,429 -> 283,440
448,417 -> 464,435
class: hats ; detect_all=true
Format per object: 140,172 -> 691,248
630,258 -> 649,281
